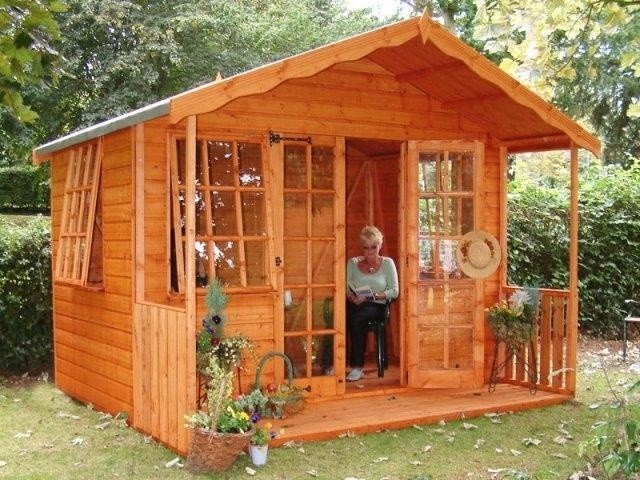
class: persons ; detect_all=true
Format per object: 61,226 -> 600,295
319,225 -> 399,383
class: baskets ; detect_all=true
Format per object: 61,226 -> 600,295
186,427 -> 255,474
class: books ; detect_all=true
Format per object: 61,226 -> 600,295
347,282 -> 375,302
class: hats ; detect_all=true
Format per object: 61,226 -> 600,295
455,229 -> 504,280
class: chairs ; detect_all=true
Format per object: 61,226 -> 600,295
320,293 -> 394,378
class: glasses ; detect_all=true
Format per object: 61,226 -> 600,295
362,243 -> 380,250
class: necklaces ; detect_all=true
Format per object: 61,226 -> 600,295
360,256 -> 381,273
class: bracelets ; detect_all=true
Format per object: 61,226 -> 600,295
375,292 -> 380,300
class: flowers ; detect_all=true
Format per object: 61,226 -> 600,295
487,285 -> 541,354
184,379 -> 312,447
194,281 -> 256,380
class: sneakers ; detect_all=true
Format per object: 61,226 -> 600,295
346,367 -> 364,381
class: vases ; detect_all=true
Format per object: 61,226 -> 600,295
249,443 -> 270,466
